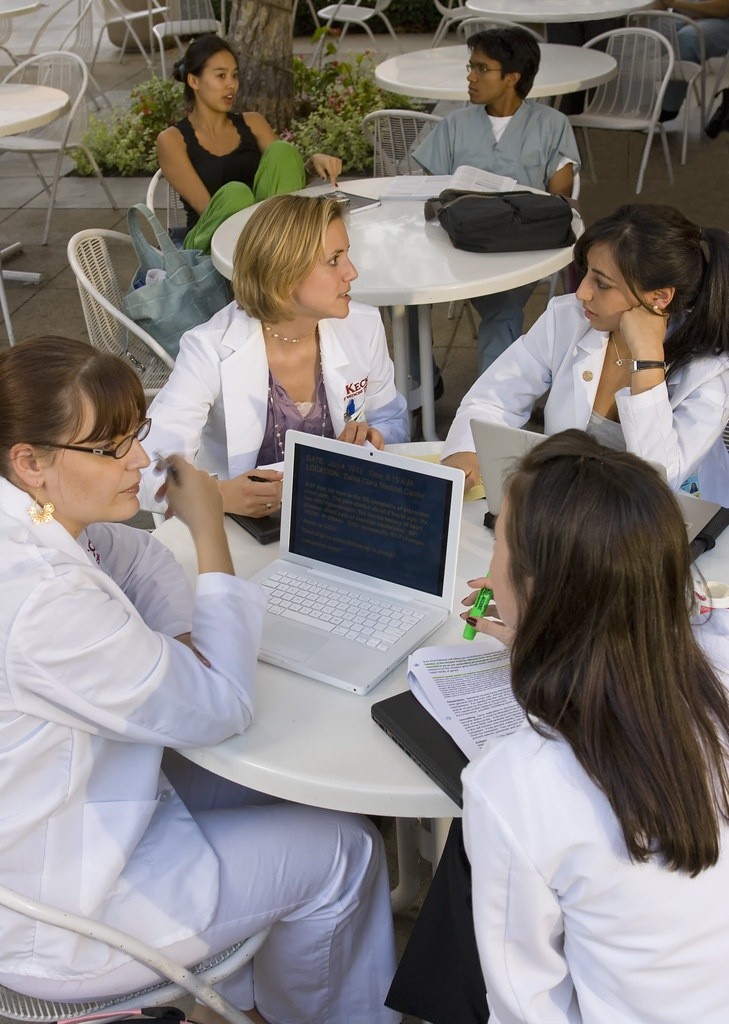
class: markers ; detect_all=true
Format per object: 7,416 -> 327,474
463,571 -> 494,641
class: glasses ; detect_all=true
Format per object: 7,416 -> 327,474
10,418 -> 151,459
467,64 -> 501,76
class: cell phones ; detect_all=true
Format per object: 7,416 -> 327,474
323,193 -> 350,202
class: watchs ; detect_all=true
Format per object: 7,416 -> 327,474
630,359 -> 666,373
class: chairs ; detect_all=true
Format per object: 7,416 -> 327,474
66,229 -> 178,395
362,109 -> 456,318
0,0 -> 729,244
0,886 -> 271,1024
146,168 -> 188,230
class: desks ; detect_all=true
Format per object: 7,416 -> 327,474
0,82 -> 72,284
374,41 -> 619,101
212,174 -> 585,442
465,0 -> 656,23
150,440 -> 729,913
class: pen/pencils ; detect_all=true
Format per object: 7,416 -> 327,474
153,451 -> 176,477
247,476 -> 268,482
347,399 -> 355,415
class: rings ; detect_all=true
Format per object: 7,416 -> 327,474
266,504 -> 271,510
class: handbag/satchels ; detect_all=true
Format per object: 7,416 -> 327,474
425,190 -> 585,253
122,203 -> 230,361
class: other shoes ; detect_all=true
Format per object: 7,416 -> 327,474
433,376 -> 444,400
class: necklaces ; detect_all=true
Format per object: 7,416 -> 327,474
266,326 -> 315,343
612,331 -> 632,366
268,352 -> 326,458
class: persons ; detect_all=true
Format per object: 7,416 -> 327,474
136,195 -> 409,518
383,428 -> 729,1024
658,0 -> 729,123
410,26 -> 581,380
156,35 -> 342,256
441,203 -> 729,585
690,483 -> 698,494
0,334 -> 397,1024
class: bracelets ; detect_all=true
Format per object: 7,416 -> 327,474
305,166 -> 317,176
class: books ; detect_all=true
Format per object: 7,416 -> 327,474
379,165 -> 517,201
323,190 -> 382,215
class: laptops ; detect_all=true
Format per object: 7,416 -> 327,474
250,429 -> 465,695
470,417 -> 722,544
370,690 -> 471,810
